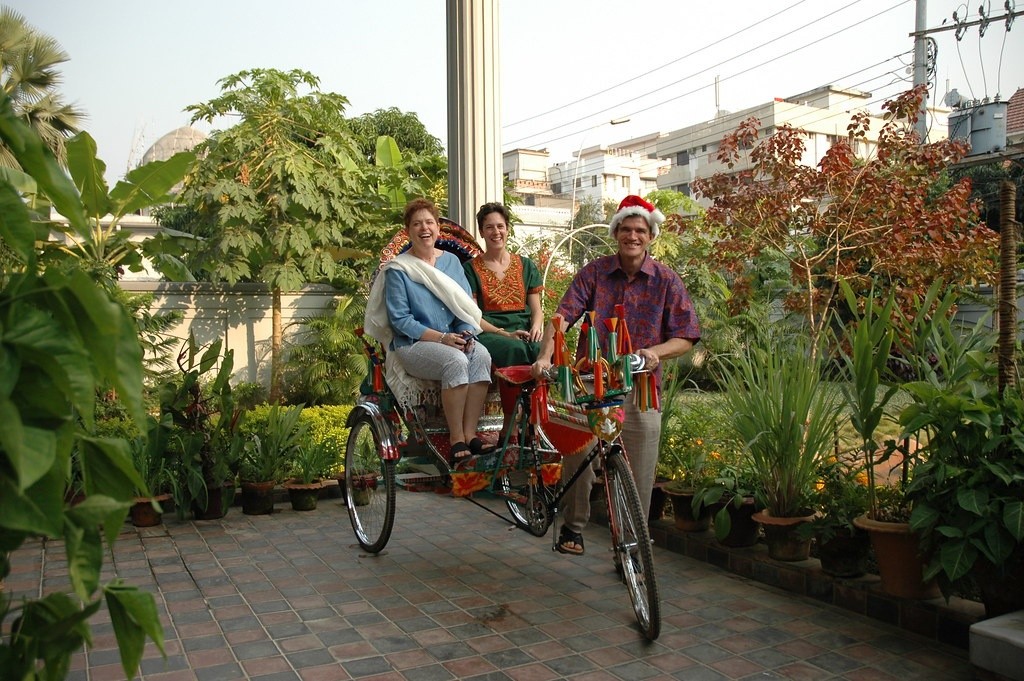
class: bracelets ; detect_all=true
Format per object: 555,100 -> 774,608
496,327 -> 504,333
438,332 -> 446,343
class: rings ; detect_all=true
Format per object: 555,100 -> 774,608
650,364 -> 653,367
453,337 -> 457,342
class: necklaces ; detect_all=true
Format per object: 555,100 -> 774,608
411,246 -> 435,261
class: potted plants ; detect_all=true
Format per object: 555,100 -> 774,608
115,426 -> 208,528
705,280 -> 856,563
837,275 -> 999,600
648,353 -> 702,521
691,470 -> 766,546
811,464 -> 886,579
333,433 -> 412,506
159,328 -> 247,520
588,467 -> 605,501
661,399 -> 720,531
897,351 -> 1024,620
238,399 -> 315,515
282,429 -> 345,511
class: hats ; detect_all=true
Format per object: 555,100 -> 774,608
608,195 -> 666,242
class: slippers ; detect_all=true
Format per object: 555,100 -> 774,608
614,551 -> 646,585
559,523 -> 584,555
465,438 -> 496,455
450,442 -> 472,463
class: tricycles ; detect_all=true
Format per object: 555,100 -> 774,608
343,218 -> 663,643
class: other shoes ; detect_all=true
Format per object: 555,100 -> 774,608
497,428 -> 519,448
525,432 -> 542,449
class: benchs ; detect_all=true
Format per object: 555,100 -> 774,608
364,217 -> 504,420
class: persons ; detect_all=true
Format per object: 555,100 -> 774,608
382,197 -> 497,463
461,202 -> 554,449
527,195 -> 704,586
